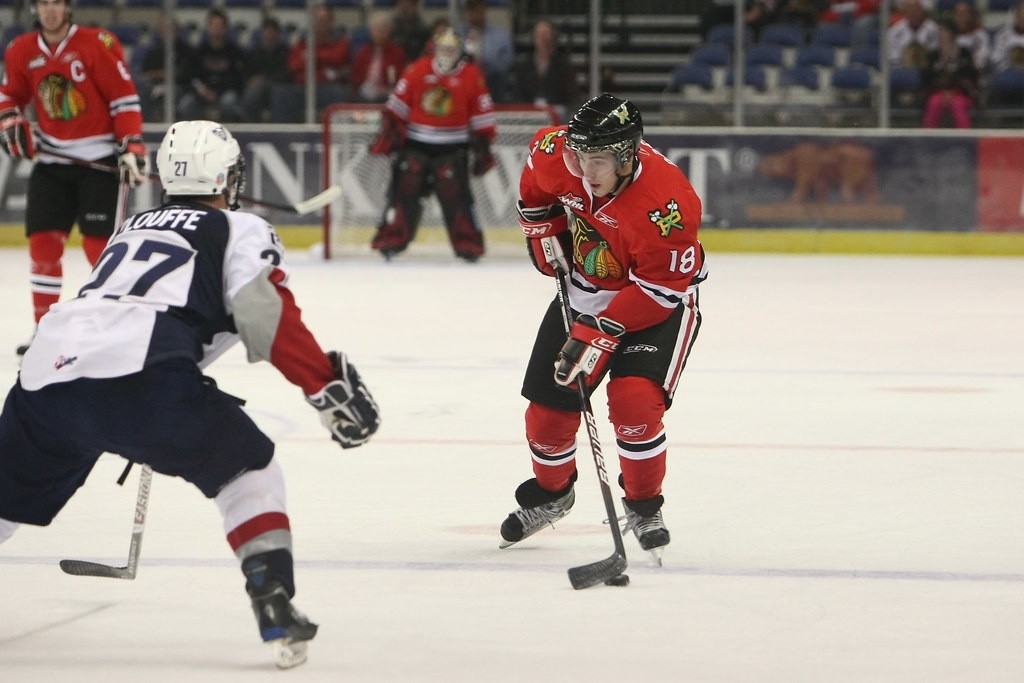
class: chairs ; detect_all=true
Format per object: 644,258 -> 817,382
659,22 -> 1024,128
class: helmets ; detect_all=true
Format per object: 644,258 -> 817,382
438,28 -> 461,70
566,92 -> 643,162
156,120 -> 242,195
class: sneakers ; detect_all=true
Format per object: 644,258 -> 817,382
240,549 -> 318,669
498,468 -> 578,550
603,473 -> 670,568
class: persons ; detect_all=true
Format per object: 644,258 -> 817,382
500,94 -> 709,552
0,119 -> 380,642
735,1 -> 1024,162
139,0 -> 579,121
369,23 -> 497,263
1,0 -> 153,355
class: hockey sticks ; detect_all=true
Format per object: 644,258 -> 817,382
552,260 -> 630,592
37,144 -> 344,217
59,462 -> 154,581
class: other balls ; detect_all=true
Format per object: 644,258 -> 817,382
604,574 -> 629,587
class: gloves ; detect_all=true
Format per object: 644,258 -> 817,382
515,199 -> 575,278
113,135 -> 152,188
369,111 -> 408,154
554,312 -> 625,392
303,347 -> 380,450
0,108 -> 37,159
472,151 -> 494,175
